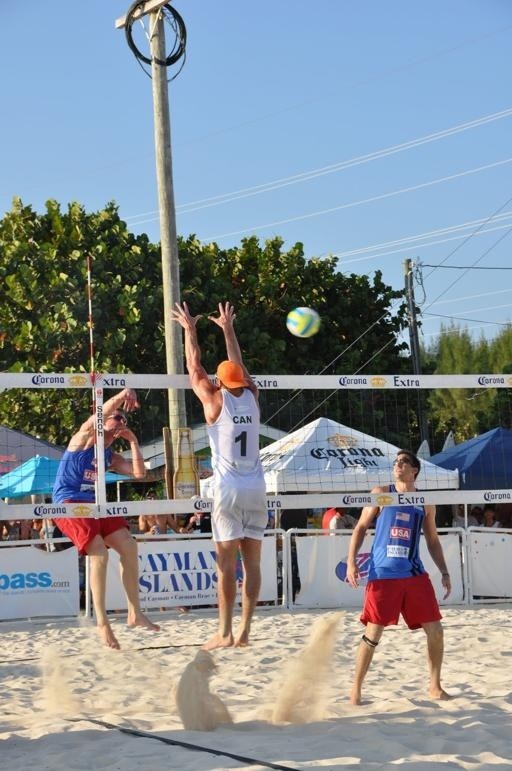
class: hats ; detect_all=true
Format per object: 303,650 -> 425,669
217,360 -> 249,388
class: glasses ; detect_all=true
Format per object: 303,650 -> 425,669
394,458 -> 411,465
108,415 -> 127,424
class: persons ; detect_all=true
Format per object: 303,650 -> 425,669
346,449 -> 451,708
168,299 -> 270,651
50,386 -> 163,651
124,512 -> 212,535
1,519 -> 65,542
268,507 -> 377,530
433,503 -> 511,530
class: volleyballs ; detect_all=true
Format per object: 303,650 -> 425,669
286,307 -> 321,338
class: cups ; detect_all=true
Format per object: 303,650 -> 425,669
152,525 -> 160,535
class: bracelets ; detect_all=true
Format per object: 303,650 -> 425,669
441,573 -> 450,577
